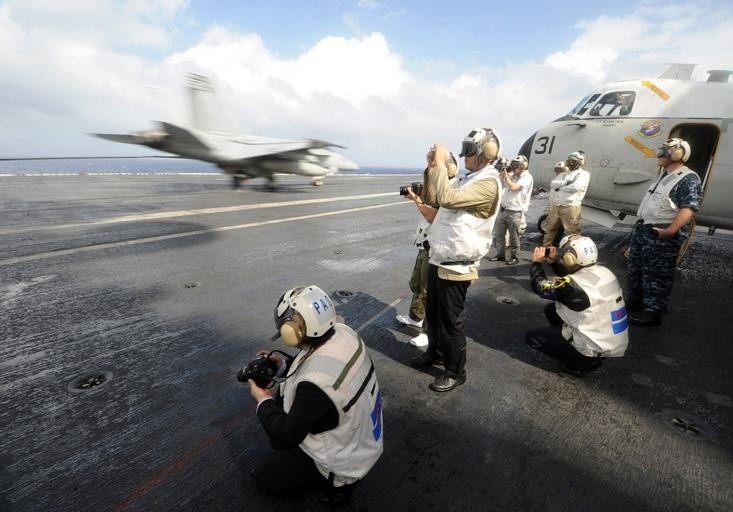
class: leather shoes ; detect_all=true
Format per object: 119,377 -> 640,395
570,357 -> 602,378
628,312 -> 662,326
411,351 -> 445,366
507,258 -> 519,265
431,374 -> 466,392
490,256 -> 505,261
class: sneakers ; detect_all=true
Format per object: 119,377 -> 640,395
396,315 -> 423,328
409,333 -> 429,346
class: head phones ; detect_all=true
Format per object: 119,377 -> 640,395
671,143 -> 684,162
563,247 -> 576,267
581,159 -> 584,165
506,157 -> 511,166
565,165 -> 569,172
483,131 -> 498,160
521,161 -> 528,170
280,312 -> 305,347
448,162 -> 456,177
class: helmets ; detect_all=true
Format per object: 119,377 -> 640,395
510,155 -> 528,173
568,151 -> 585,170
270,285 -> 335,347
553,162 -> 569,174
459,128 -> 500,166
557,234 -> 598,267
657,138 -> 691,163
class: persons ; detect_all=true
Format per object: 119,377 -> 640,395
249,284 -> 384,508
542,152 -> 590,247
624,137 -> 702,326
490,155 -> 533,265
549,161 -> 570,250
525,234 -> 629,378
410,128 -> 502,392
493,157 -> 527,248
396,152 -> 459,347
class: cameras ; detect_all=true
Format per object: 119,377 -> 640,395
430,146 -> 436,153
545,248 -> 550,257
237,356 -> 279,389
400,182 -> 422,196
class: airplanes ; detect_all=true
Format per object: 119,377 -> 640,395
511,77 -> 731,251
91,72 -> 365,193
